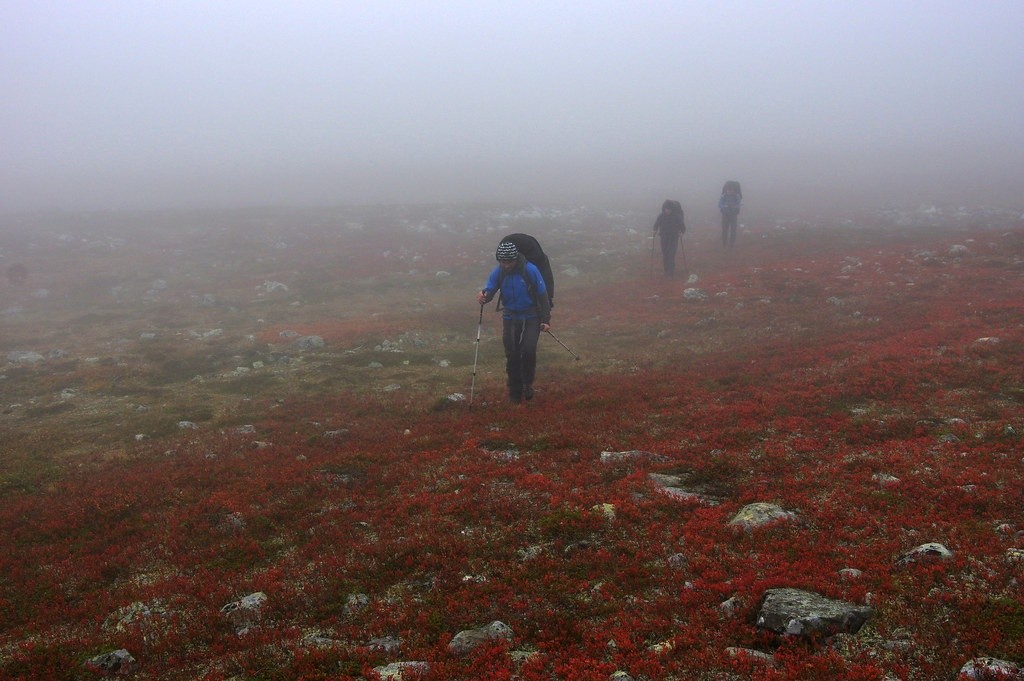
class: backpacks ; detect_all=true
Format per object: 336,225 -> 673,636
496,233 -> 553,307
663,201 -> 683,220
723,180 -> 742,202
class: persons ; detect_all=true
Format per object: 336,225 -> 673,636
718,184 -> 741,249
653,200 -> 686,275
476,241 -> 551,405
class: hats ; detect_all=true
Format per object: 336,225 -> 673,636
497,241 -> 519,259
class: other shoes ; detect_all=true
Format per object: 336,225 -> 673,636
523,383 -> 533,400
510,383 -> 522,404
